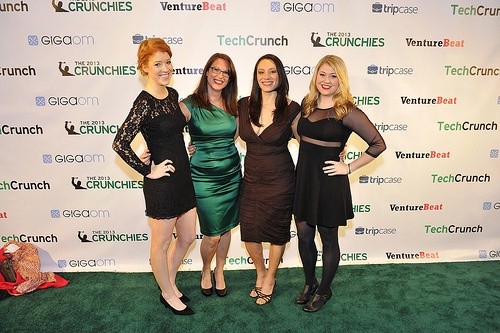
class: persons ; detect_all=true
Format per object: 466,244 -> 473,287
112,38 -> 196,316
140,53 -> 242,297
187,53 -> 347,305
292,55 -> 387,313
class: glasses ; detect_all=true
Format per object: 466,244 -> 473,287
210,65 -> 231,78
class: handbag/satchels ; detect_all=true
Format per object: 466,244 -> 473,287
0,253 -> 17,282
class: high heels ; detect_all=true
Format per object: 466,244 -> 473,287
250,286 -> 262,298
303,287 -> 332,312
296,278 -> 319,304
213,267 -> 227,297
158,284 -> 189,304
257,278 -> 277,305
160,294 -> 195,315
200,270 -> 213,296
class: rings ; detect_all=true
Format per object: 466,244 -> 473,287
167,165 -> 168,168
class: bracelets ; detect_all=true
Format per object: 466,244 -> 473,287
347,164 -> 351,174
